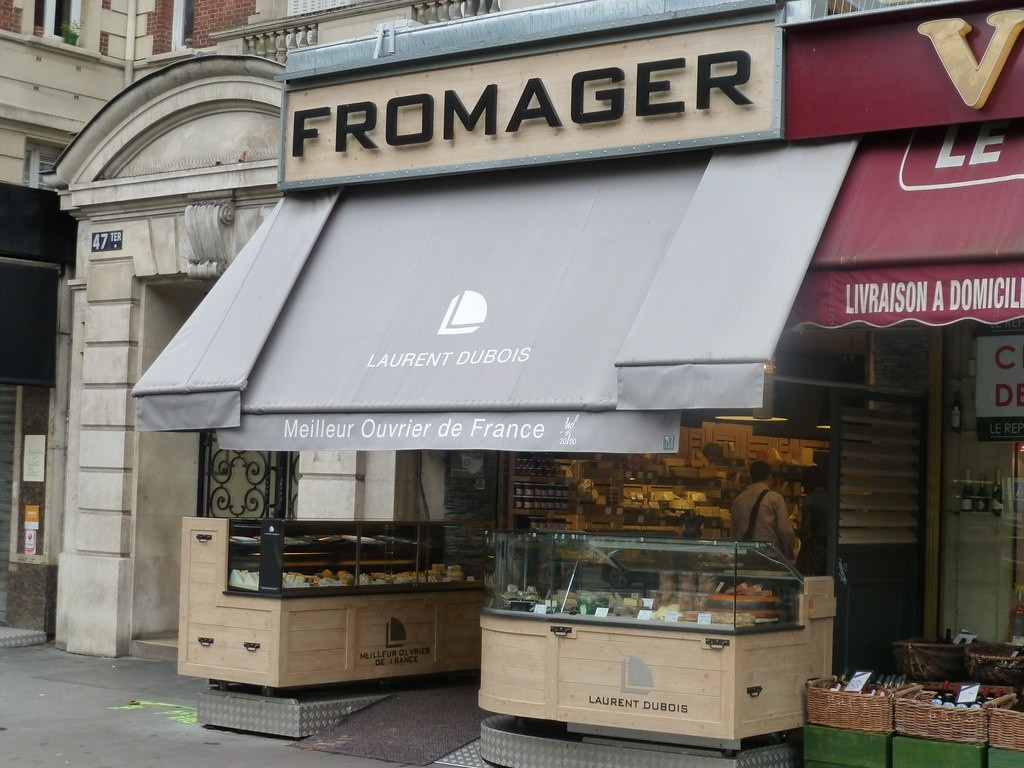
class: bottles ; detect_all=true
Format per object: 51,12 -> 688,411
827,667 -> 907,698
975,474 -> 988,512
991,467 -> 1002,512
941,628 -> 953,644
515,452 -> 561,477
930,681 -> 1004,712
960,468 -> 973,511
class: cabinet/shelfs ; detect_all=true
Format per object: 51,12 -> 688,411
508,450 -> 573,529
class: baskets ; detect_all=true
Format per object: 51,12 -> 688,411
806,675 -> 1023,752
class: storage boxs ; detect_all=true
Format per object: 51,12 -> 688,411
800,726 -> 1024,768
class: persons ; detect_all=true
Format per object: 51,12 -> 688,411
796,466 -> 827,576
730,461 -> 795,556
690,441 -> 723,467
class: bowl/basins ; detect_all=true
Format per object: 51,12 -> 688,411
575,590 -> 611,615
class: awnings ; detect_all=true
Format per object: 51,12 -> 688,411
132,117 -> 1023,452
